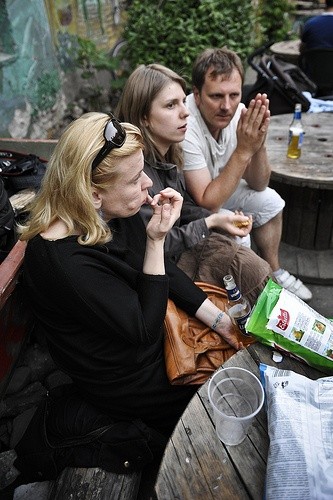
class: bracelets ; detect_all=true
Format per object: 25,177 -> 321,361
211,312 -> 225,331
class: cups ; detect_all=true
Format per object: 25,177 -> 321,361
209,367 -> 264,445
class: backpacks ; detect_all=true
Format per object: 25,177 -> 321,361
0,149 -> 46,188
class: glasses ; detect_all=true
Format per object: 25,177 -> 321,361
92,112 -> 127,168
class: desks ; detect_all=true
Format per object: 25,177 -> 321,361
264,111 -> 333,251
269,41 -> 303,68
154,342 -> 333,500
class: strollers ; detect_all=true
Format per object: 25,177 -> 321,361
246,40 -> 333,116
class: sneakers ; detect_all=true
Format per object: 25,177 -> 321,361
276,270 -> 313,300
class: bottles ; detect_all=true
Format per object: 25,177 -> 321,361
224,274 -> 255,343
287,103 -> 303,158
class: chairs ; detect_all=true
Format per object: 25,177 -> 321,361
297,49 -> 333,96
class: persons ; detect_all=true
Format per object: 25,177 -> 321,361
300,0 -> 333,95
14,112 -> 260,475
168,47 -> 313,301
113,64 -> 279,308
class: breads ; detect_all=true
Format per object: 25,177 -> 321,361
234,221 -> 249,228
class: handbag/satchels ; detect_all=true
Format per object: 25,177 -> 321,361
163,282 -> 256,386
